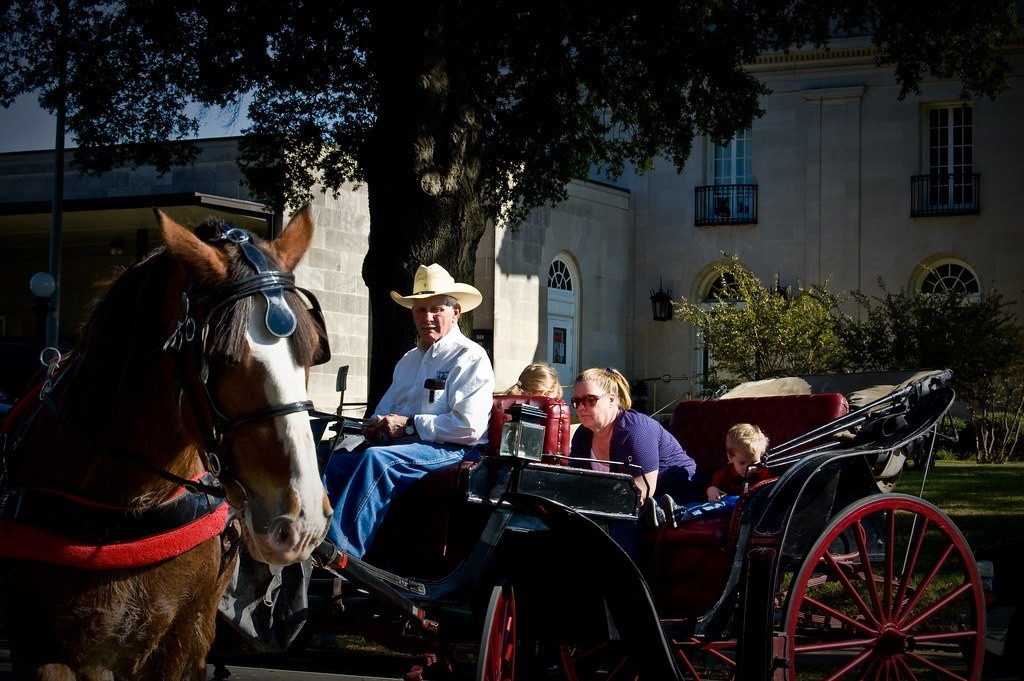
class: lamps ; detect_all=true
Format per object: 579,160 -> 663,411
648,272 -> 672,320
799,84 -> 866,104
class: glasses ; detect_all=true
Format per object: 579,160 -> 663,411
571,395 -> 599,409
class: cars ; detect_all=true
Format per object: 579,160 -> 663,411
958,538 -> 1024,681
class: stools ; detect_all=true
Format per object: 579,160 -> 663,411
393,453 -> 494,573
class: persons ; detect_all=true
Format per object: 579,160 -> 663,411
708,423 -> 774,503
505,362 -> 563,399
572,368 -> 696,505
322,263 -> 495,559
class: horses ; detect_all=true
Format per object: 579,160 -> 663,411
0,199 -> 333,681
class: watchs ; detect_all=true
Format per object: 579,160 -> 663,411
405,417 -> 415,436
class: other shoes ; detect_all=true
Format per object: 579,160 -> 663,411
661,494 -> 685,529
646,496 -> 666,527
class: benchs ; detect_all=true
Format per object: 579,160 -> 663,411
636,392 -> 851,546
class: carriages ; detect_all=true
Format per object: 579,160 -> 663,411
0,203 -> 985,681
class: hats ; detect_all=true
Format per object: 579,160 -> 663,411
390,263 -> 483,313
